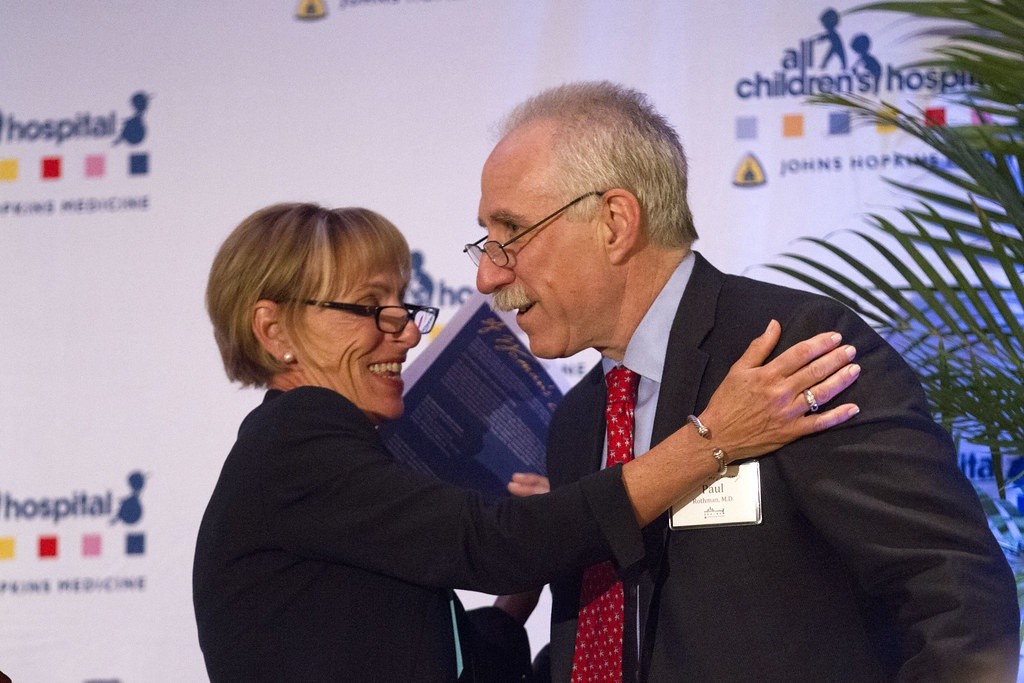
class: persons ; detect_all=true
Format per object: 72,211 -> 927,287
193,199 -> 862,683
425,79 -> 1022,683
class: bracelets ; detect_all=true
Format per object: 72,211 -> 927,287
684,413 -> 729,477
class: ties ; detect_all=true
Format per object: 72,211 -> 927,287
571,367 -> 638,683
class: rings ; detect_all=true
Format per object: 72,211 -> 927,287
800,390 -> 819,414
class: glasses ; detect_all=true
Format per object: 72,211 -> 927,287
276,297 -> 439,335
463,191 -> 604,267
